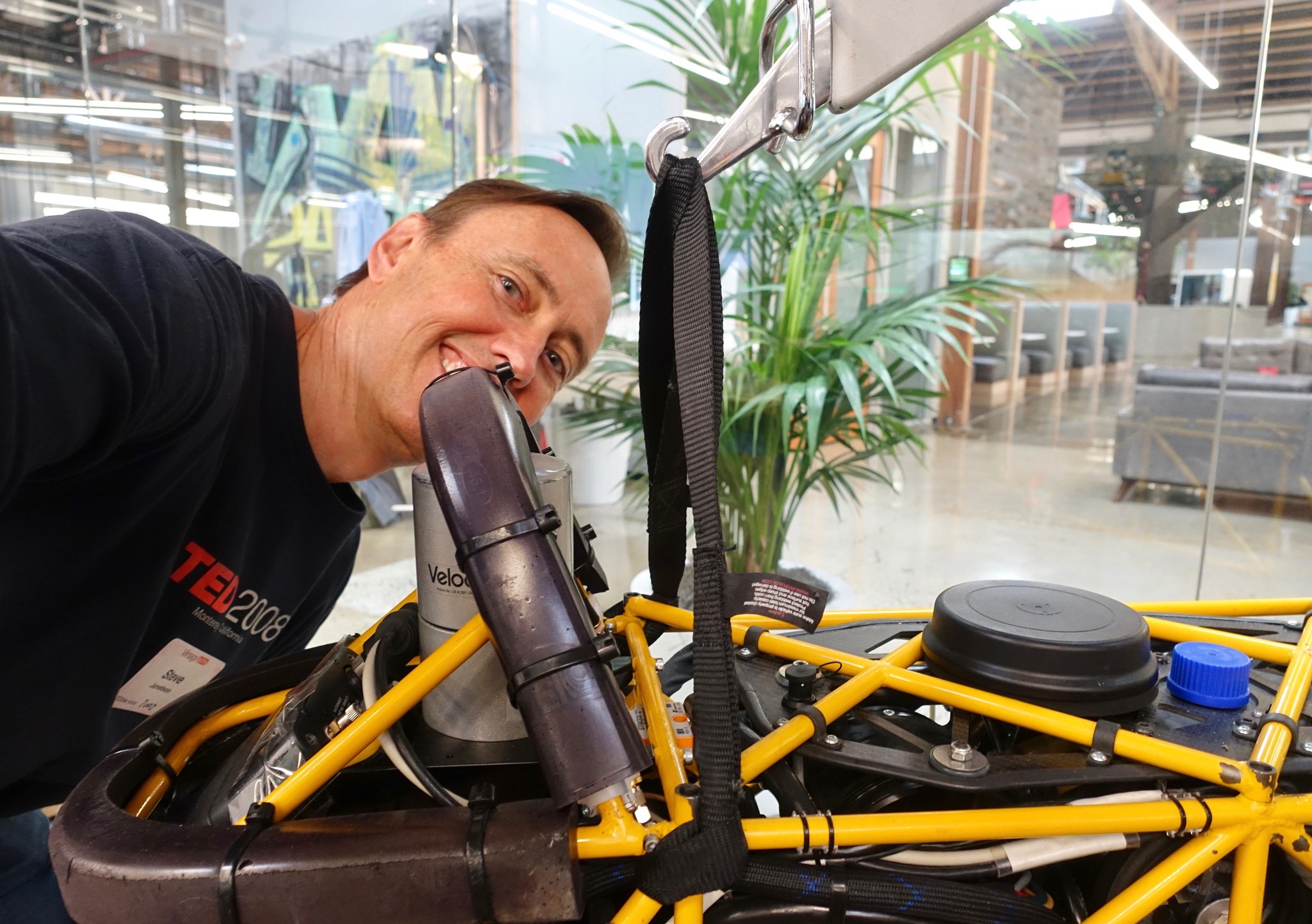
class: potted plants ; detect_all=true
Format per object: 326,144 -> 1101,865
484,126 -> 656,503
604,1 -> 1092,779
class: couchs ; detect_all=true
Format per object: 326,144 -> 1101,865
1112,338 -> 1312,515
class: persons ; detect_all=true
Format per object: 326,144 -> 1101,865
0,177 -> 629,924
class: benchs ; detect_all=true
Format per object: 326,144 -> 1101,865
970,303 -> 1136,419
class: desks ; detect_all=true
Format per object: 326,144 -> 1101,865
972,334 -> 996,346
1022,333 -> 1047,341
1103,328 -> 1119,334
1066,329 -> 1087,338
